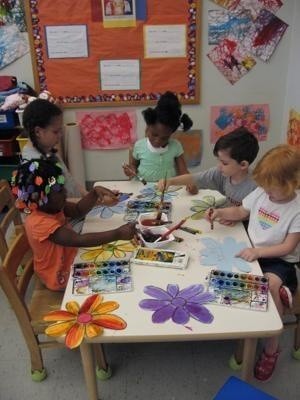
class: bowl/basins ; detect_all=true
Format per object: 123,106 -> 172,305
140,226 -> 176,250
138,210 -> 170,228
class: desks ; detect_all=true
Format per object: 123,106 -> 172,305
56,180 -> 283,400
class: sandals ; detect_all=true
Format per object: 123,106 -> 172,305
253,342 -> 281,381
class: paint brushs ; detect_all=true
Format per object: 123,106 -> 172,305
209,208 -> 213,230
123,163 -> 147,186
153,220 -> 173,225
152,218 -> 185,242
156,174 -> 167,220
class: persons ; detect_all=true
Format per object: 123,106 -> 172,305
22,98 -> 118,206
206,146 -> 300,381
12,158 -> 137,291
159,125 -> 257,227
121,90 -> 199,194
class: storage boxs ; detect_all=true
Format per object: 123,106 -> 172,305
0,109 -> 19,129
0,138 -> 13,158
0,87 -> 20,107
16,133 -> 30,152
0,165 -> 18,182
15,107 -> 25,127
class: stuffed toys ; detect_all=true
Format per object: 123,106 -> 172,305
37,90 -> 58,109
2,93 -> 36,111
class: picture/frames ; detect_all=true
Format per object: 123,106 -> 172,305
24,0 -> 203,109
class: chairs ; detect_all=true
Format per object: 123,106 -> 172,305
1,185 -> 113,382
228,266 -> 300,371
